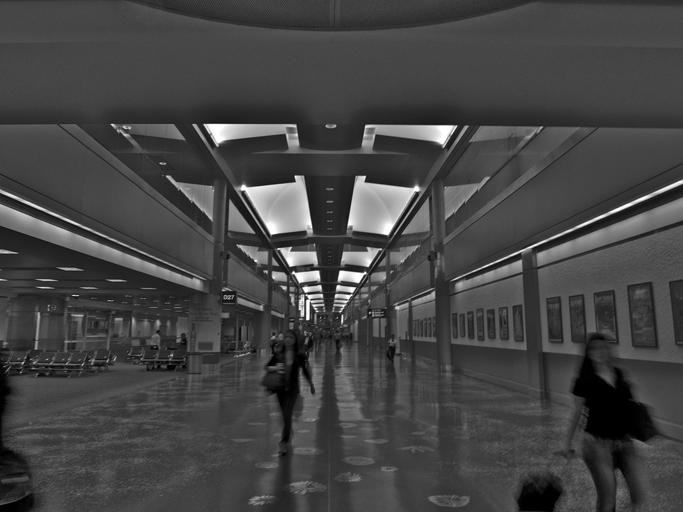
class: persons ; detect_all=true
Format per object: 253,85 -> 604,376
178,332 -> 187,346
560,331 -> 648,512
499,307 -> 507,337
384,333 -> 397,362
244,340 -> 249,349
0,362 -> 34,512
512,466 -> 563,511
248,341 -> 256,354
261,327 -> 316,458
150,330 -> 161,370
267,323 -> 352,356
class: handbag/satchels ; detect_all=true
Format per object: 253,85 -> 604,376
628,399 -> 658,442
260,372 -> 284,388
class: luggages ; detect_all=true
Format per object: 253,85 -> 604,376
517,449 -> 575,512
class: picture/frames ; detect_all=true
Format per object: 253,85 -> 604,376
591,289 -> 620,345
411,303 -> 524,344
666,279 -> 681,346
544,295 -> 563,346
567,293 -> 587,345
626,280 -> 658,349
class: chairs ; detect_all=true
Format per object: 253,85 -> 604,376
1,334 -> 242,381
